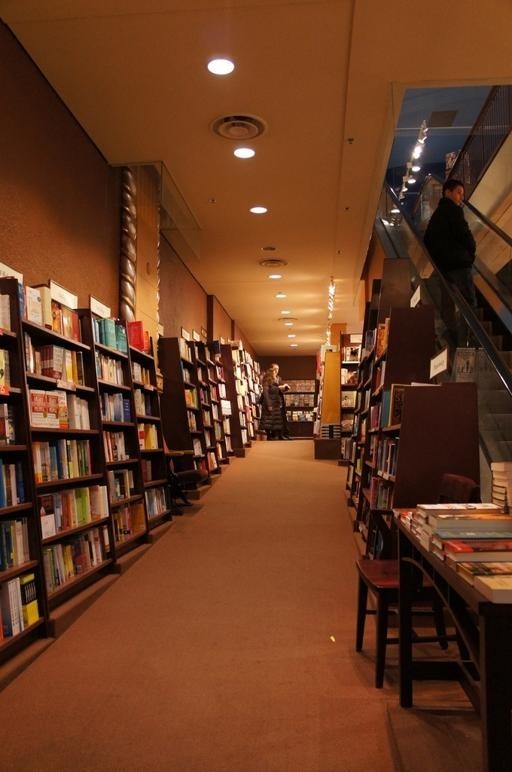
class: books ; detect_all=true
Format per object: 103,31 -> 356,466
342,314 -> 445,557
280,379 -> 313,422
20,283 -> 113,594
320,422 -> 342,440
0,262 -> 40,647
313,362 -> 324,436
395,458 -> 512,610
177,338 -> 261,472
93,317 -> 166,546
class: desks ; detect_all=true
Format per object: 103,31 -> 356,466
391,508 -> 512,772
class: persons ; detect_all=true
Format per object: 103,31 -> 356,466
421,177 -> 476,349
257,362 -> 294,441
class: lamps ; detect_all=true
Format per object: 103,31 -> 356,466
326,275 -> 336,348
284,310 -> 290,315
284,322 -> 294,325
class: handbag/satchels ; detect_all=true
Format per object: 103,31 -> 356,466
258,393 -> 264,404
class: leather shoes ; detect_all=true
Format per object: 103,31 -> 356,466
267,435 -> 292,440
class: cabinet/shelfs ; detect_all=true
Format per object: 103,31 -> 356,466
1,276 -> 173,664
156,326 -> 264,501
344,257 -> 480,615
282,331 -> 363,460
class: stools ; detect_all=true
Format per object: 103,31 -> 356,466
356,560 -> 480,689
